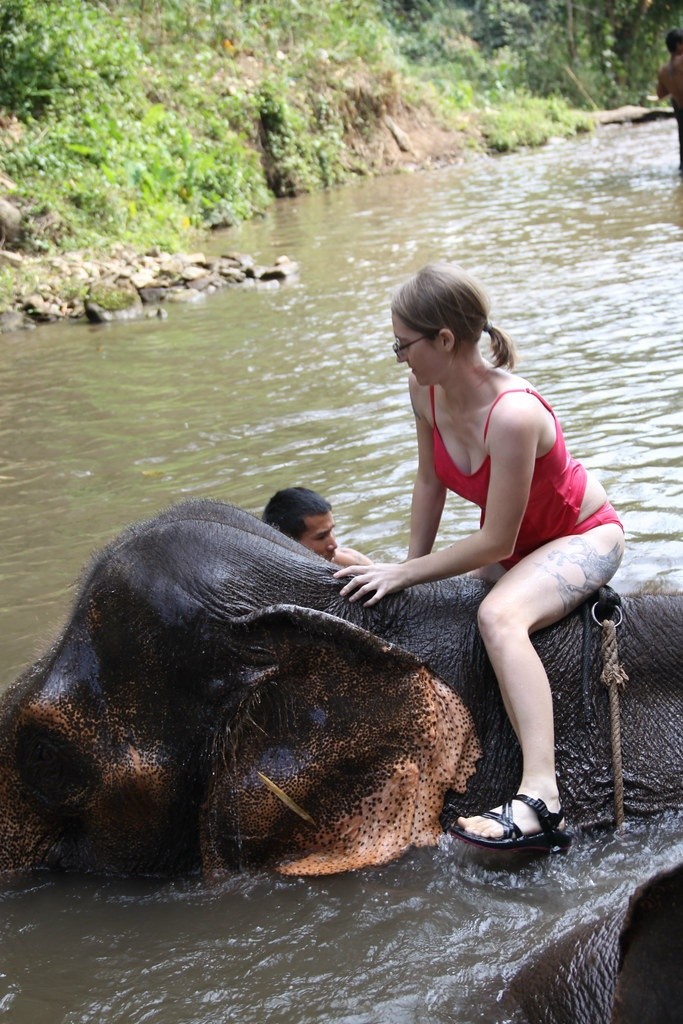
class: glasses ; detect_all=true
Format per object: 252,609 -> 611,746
392,335 -> 425,359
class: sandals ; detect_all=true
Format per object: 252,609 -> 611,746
450,794 -> 572,853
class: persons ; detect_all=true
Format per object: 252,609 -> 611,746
260,487 -> 374,567
334,262 -> 628,853
656,29 -> 683,174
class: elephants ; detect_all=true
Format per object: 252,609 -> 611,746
0,500 -> 682,1020
